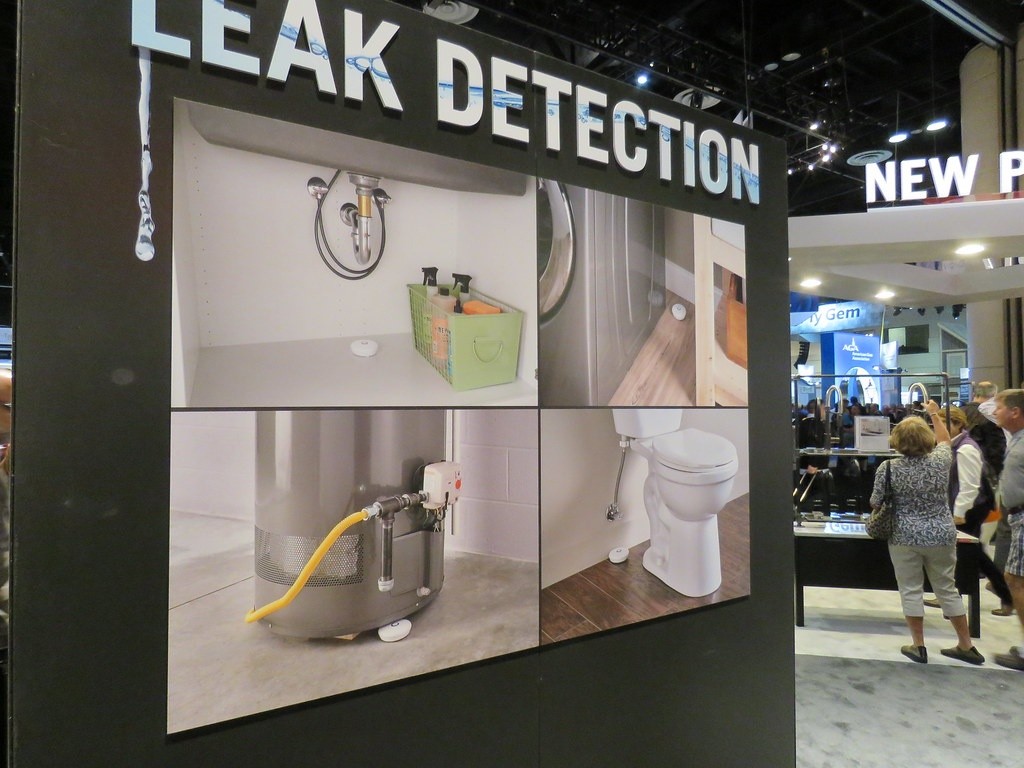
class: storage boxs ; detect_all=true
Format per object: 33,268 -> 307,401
406,282 -> 524,389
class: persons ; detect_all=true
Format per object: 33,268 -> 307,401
792,396 -> 933,521
978,388 -> 1024,672
923,405 -> 1014,615
958,380 -> 1012,550
869,399 -> 985,665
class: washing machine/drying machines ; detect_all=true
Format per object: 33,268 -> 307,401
536,176 -> 667,406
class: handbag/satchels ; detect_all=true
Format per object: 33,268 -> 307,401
865,460 -> 895,539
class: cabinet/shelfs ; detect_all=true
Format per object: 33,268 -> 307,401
791,373 -> 982,638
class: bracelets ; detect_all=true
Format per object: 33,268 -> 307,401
930,413 -> 937,416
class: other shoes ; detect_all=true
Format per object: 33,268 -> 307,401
992,604 -> 1012,616
924,598 -> 940,608
902,645 -> 927,663
996,646 -> 1024,670
940,644 -> 984,664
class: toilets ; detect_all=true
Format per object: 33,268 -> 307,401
612,409 -> 738,598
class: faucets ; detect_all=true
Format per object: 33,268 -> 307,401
824,384 -> 843,454
908,382 -> 928,417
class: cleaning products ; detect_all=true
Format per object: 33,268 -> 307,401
423,267 -> 500,383
